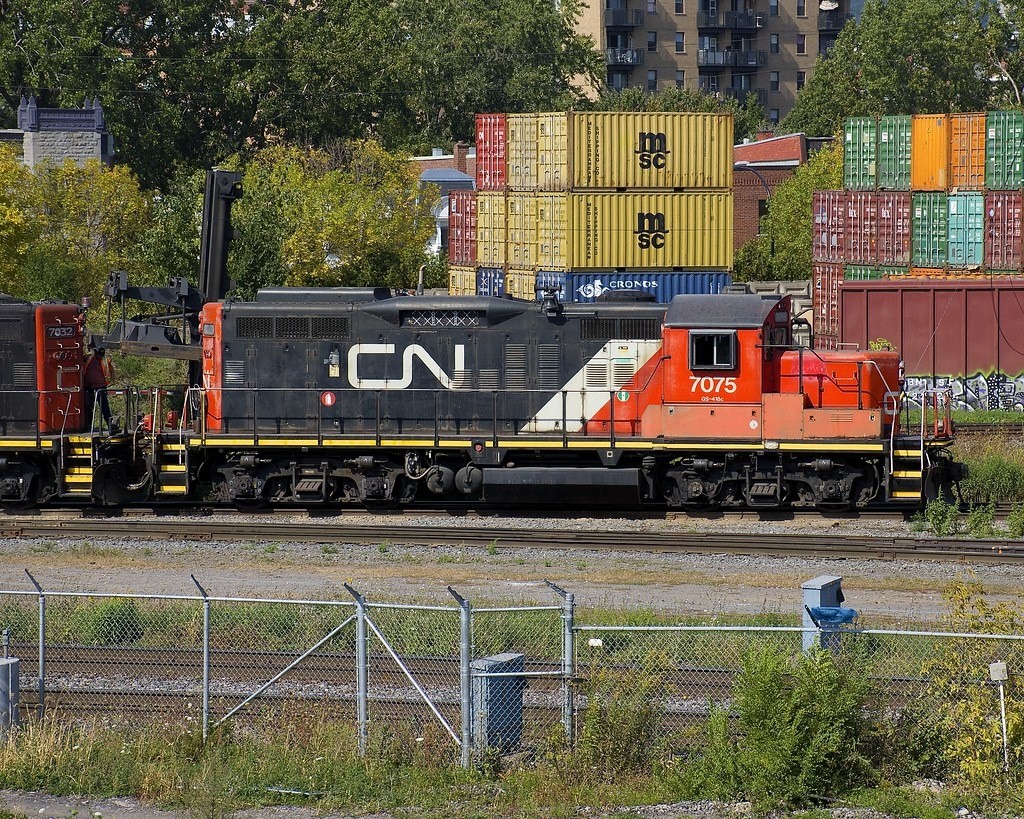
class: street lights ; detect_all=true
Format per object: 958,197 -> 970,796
734,165 -> 775,256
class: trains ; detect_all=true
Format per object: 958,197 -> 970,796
1,286 -> 969,511
